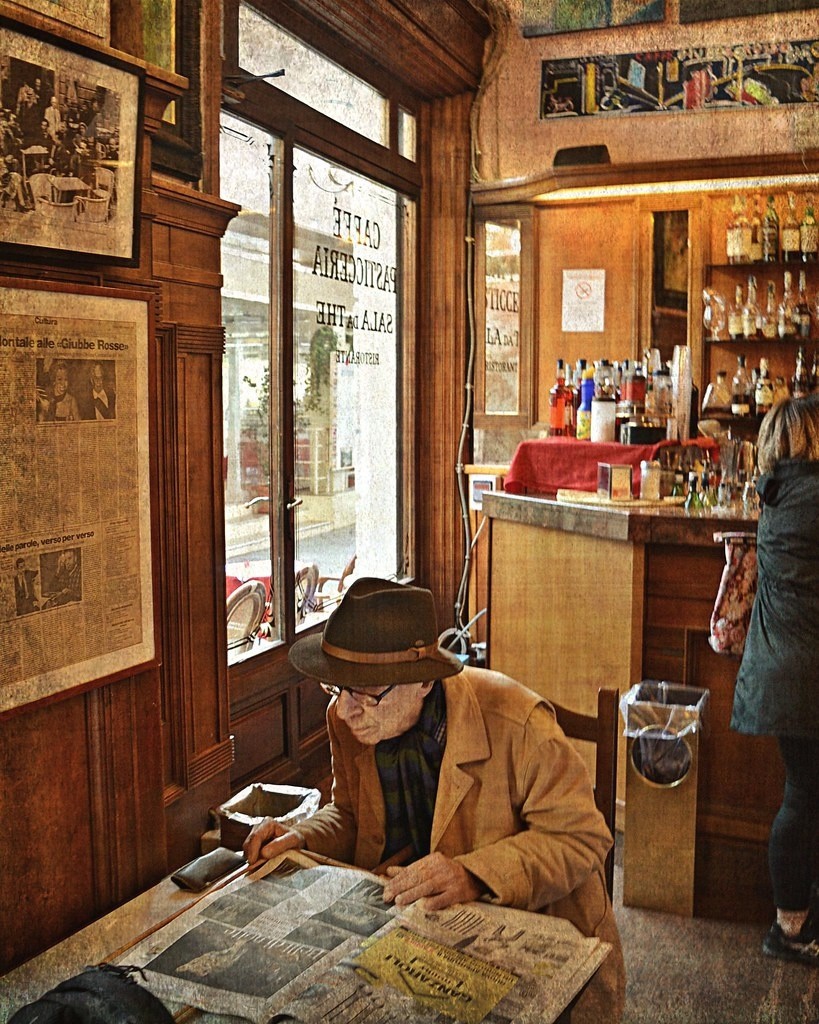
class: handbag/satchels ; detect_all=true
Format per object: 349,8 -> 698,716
709,530 -> 758,656
7,964 -> 176,1024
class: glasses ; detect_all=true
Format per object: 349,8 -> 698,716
320,680 -> 397,707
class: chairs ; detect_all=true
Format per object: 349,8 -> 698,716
468,658 -> 619,906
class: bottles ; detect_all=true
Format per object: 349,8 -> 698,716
791,346 -> 810,397
752,367 -> 761,415
755,358 -> 774,416
810,349 -> 819,395
743,275 -> 762,339
813,291 -> 819,338
671,453 -> 760,517
797,270 -> 813,339
725,191 -> 819,266
549,347 -> 674,445
731,355 -> 752,416
761,280 -> 779,339
773,376 -> 789,407
779,271 -> 797,339
728,284 -> 745,340
702,371 -> 732,418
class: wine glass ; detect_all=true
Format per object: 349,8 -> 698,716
703,304 -> 726,340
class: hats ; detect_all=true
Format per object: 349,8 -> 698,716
288,578 -> 463,686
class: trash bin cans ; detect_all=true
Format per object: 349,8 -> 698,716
623,683 -> 713,919
215,782 -> 321,853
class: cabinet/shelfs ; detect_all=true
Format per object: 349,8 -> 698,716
689,186 -> 819,425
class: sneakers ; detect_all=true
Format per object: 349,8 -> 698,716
763,908 -> 819,966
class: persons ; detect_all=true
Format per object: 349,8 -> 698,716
730,396 -> 819,965
83,364 -> 116,420
243,577 -> 627,1024
50,550 -> 82,601
0,65 -> 119,213
36,360 -> 81,422
14,558 -> 40,617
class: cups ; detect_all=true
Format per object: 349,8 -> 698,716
702,287 -> 726,304
639,461 -> 660,502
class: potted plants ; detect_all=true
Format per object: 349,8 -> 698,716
242,368 -> 270,511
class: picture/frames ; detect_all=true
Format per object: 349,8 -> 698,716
0,14 -> 146,269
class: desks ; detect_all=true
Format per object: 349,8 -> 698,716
0,852 -> 615,1024
